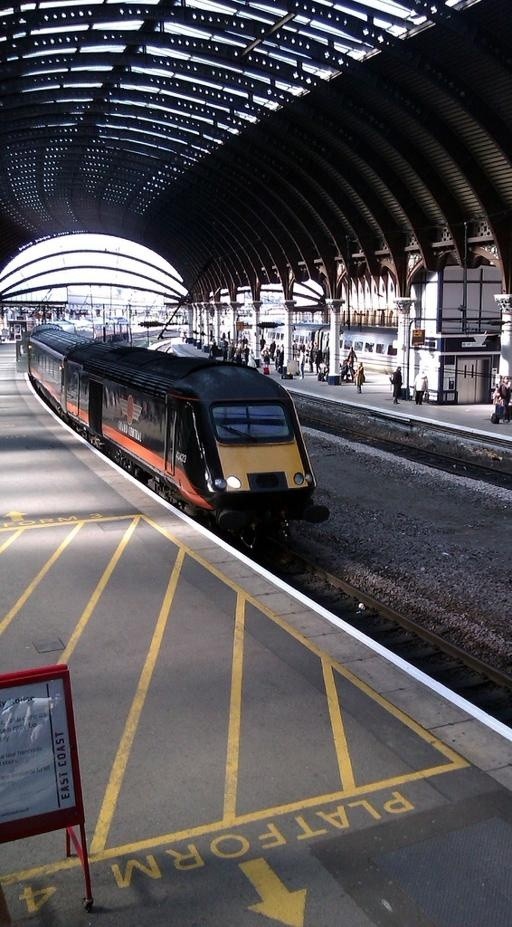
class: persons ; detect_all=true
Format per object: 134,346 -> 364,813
178,323 -> 366,395
413,370 -> 428,405
494,375 -> 511,422
389,365 -> 403,404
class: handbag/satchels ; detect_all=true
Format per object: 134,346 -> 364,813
491,414 -> 500,425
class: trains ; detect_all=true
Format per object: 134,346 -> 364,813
240,321 -> 398,377
17,317 -> 333,563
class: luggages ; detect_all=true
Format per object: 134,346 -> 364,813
263,367 -> 269,375
